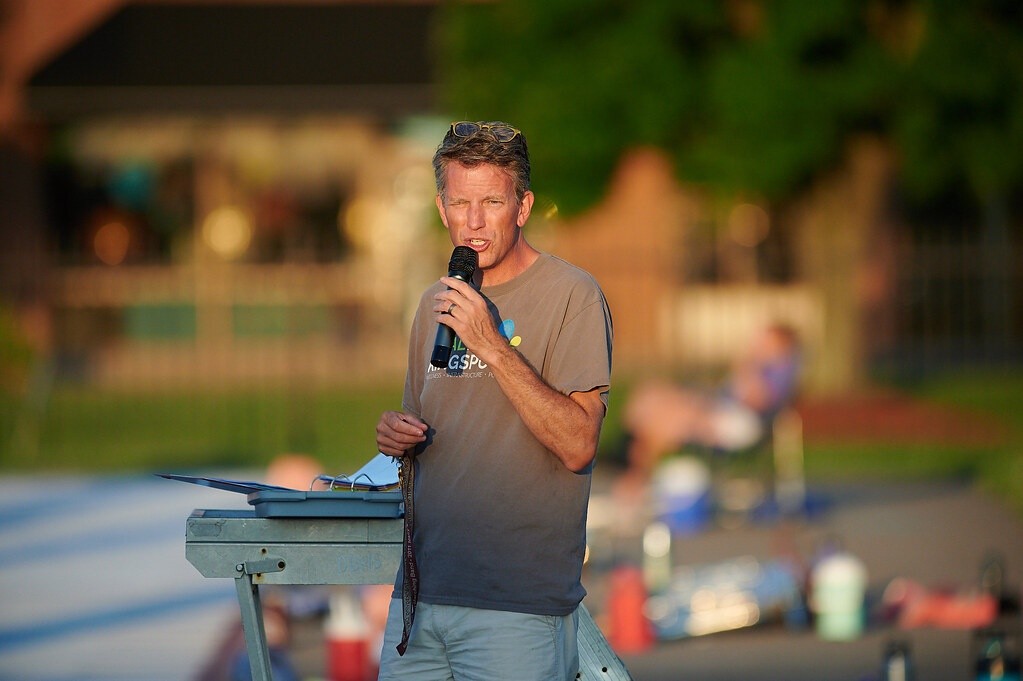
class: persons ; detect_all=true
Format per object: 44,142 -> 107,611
375,119 -> 615,680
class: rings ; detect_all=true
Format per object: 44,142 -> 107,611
448,304 -> 457,314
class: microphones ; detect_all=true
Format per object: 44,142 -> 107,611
431,246 -> 477,368
